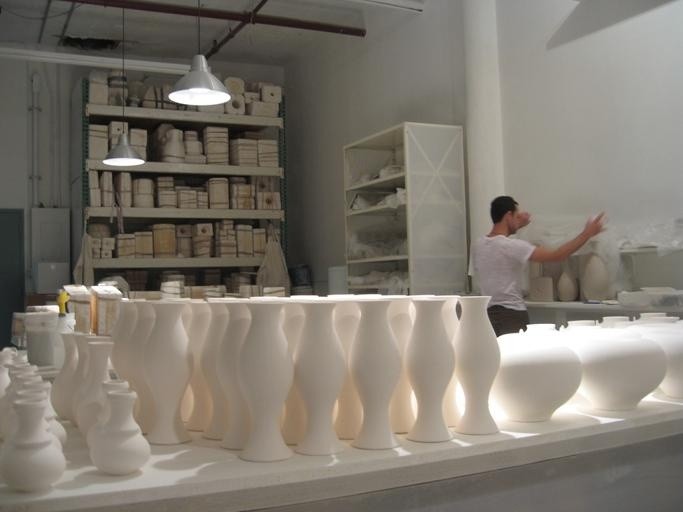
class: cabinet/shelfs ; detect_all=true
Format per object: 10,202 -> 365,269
80,101 -> 284,285
341,121 -> 469,299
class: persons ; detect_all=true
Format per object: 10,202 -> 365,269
468,194 -> 608,337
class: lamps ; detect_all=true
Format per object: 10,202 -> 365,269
100,2 -> 232,167
